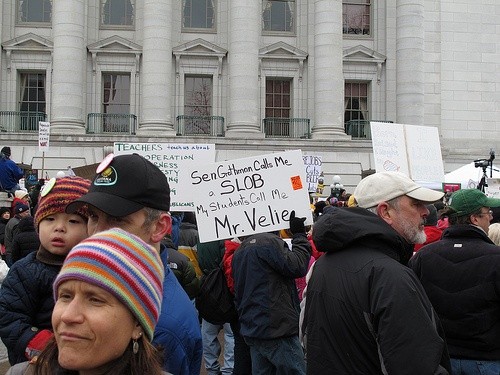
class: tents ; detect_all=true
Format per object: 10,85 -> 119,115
444,158 -> 500,189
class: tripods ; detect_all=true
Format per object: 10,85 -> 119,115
477,174 -> 487,194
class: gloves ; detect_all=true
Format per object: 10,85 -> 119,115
289,210 -> 306,234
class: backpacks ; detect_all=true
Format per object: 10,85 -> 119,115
195,260 -> 238,326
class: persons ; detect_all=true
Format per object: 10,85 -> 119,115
0,146 -> 24,195
0,153 -> 202,375
179,172 -> 500,375
338,188 -> 349,201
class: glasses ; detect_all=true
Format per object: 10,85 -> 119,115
468,210 -> 493,219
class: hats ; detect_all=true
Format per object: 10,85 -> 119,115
354,171 -> 445,209
52,227 -> 165,344
1,147 -> 11,156
65,153 -> 170,217
34,175 -> 93,235
15,190 -> 29,199
340,189 -> 345,194
14,202 -> 29,214
446,189 -> 500,218
314,201 -> 326,213
